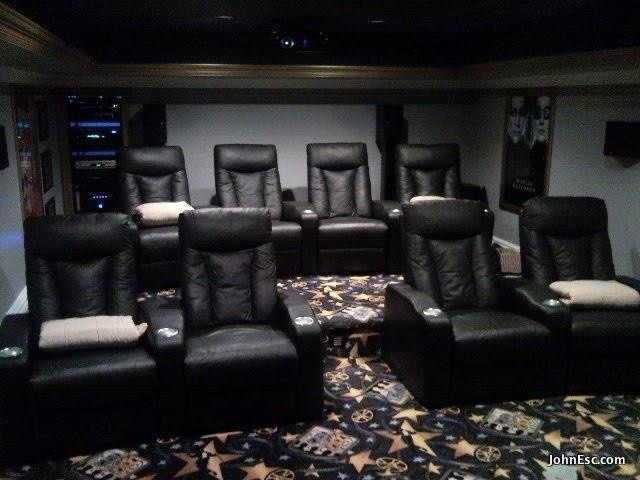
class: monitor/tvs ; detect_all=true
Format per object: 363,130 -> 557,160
68,119 -> 123,160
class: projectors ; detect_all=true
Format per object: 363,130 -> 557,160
269,27 -> 329,55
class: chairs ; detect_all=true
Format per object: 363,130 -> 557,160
378,195 -> 637,414
2,203 -> 329,454
120,141 -> 493,294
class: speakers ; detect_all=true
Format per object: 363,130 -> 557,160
602,121 -> 640,159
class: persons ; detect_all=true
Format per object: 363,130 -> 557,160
529,94 -> 551,195
504,92 -> 529,207
